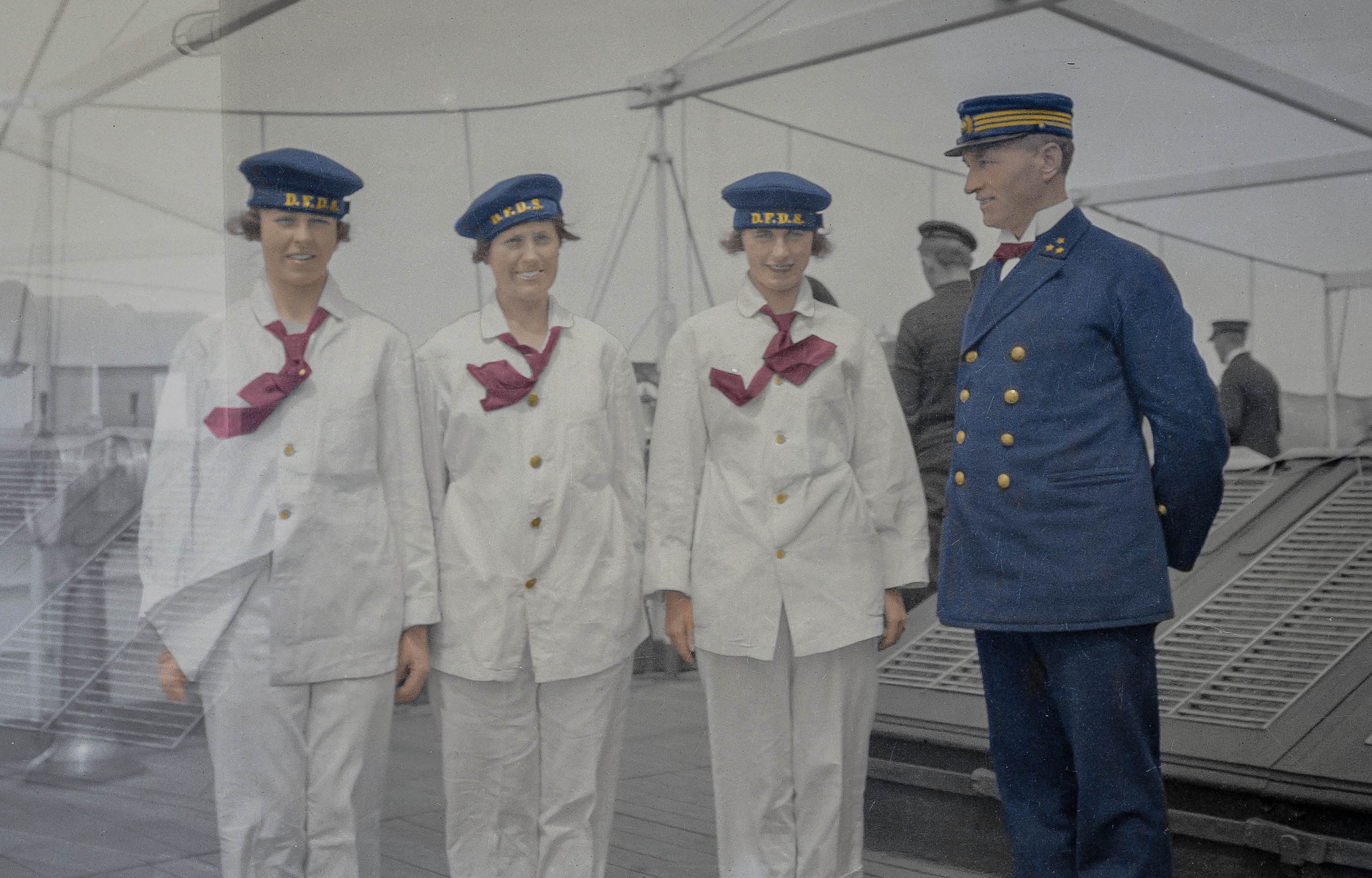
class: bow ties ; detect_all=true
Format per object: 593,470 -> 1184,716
996,241 -> 1033,262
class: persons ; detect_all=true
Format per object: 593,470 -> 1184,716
639,172 -> 931,878
897,220 -> 978,595
1207,321 -> 1282,471
935,94 -> 1229,878
414,173 -> 669,878
137,145 -> 442,878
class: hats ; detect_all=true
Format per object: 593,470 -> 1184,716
455,173 -> 563,242
944,92 -> 1073,157
237,146 -> 364,214
719,171 -> 830,231
918,220 -> 976,252
1208,321 -> 1248,341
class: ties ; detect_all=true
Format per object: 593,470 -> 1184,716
467,327 -> 562,411
710,305 -> 837,405
204,306 -> 331,438
